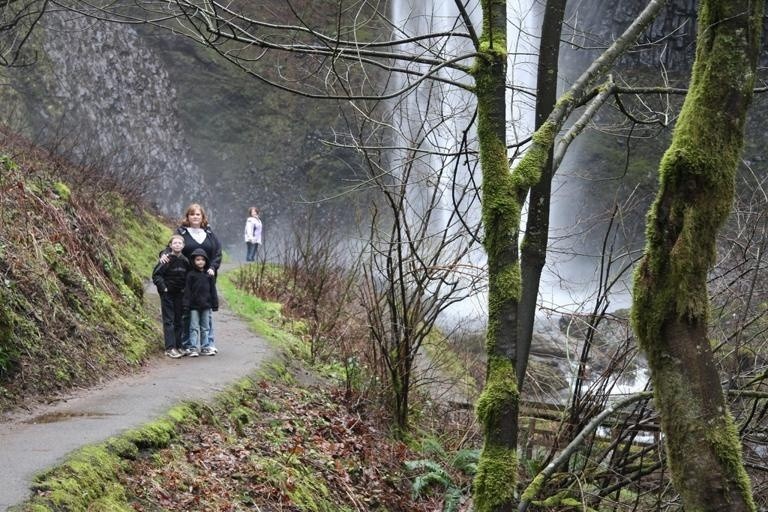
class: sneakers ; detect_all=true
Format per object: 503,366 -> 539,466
164,346 -> 217,359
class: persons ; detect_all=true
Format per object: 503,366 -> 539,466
153,234 -> 191,358
159,203 -> 222,354
183,248 -> 218,356
244,207 -> 262,264
